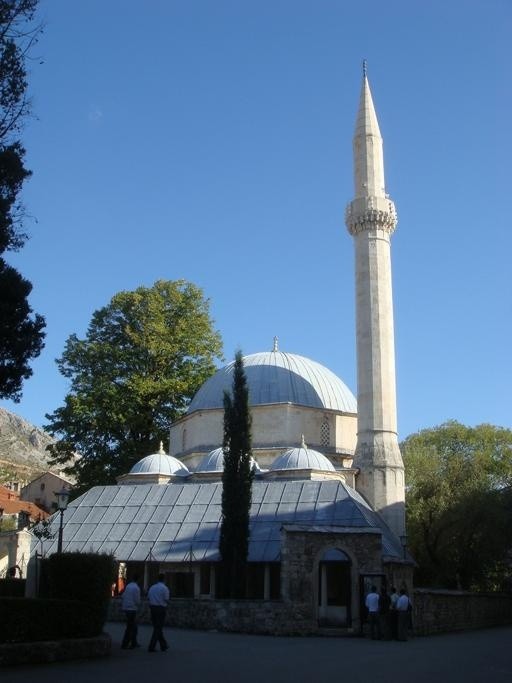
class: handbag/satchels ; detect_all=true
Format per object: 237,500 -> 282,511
406,596 -> 412,614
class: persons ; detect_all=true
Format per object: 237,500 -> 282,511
387,586 -> 400,638
119,571 -> 144,649
393,588 -> 413,642
146,572 -> 171,652
366,583 -> 383,639
377,586 -> 391,641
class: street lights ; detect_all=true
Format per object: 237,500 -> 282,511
53,484 -> 69,552
398,530 -> 410,560
